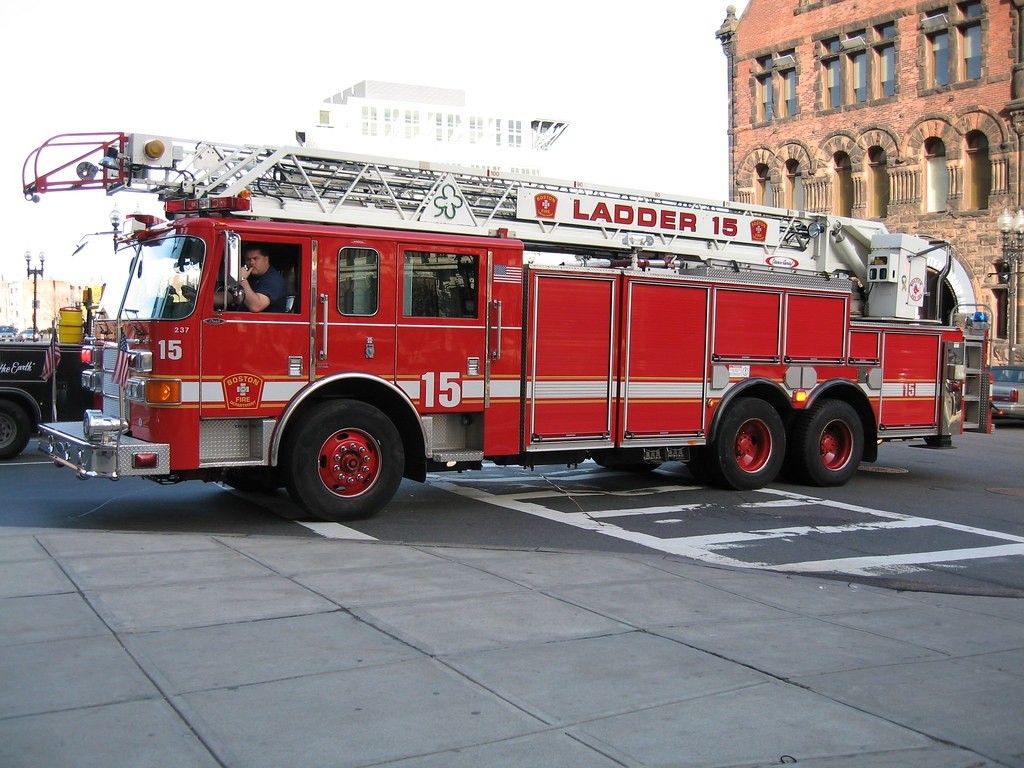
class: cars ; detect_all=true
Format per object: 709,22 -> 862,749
986,365 -> 1024,418
0,325 -> 40,342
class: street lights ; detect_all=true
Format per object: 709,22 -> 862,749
998,209 -> 1024,364
24,250 -> 45,343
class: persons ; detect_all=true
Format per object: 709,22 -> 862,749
184,246 -> 285,313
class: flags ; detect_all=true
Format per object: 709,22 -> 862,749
39,329 -> 61,380
111,331 -> 129,391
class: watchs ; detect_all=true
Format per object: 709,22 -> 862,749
239,277 -> 247,282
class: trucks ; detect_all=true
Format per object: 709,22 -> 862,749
0,288 -> 96,460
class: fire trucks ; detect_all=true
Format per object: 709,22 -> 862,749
23,131 -> 995,521
